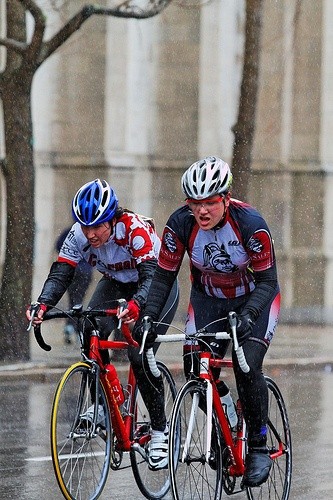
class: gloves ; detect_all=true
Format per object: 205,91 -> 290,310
227,309 -> 256,347
132,319 -> 157,352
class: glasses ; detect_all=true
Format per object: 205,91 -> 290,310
186,197 -> 224,214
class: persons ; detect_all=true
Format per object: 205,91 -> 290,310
110,321 -> 135,363
129,155 -> 283,489
26,177 -> 180,471
54,226 -> 94,344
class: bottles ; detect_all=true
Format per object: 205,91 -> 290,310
217,381 -> 238,427
106,359 -> 124,407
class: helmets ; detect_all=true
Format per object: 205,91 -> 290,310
71,178 -> 119,226
181,156 -> 233,200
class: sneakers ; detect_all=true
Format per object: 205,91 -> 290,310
243,453 -> 272,487
73,403 -> 106,430
208,418 -> 232,470
147,425 -> 170,471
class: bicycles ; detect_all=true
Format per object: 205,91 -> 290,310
26,298 -> 182,500
135,311 -> 292,500
